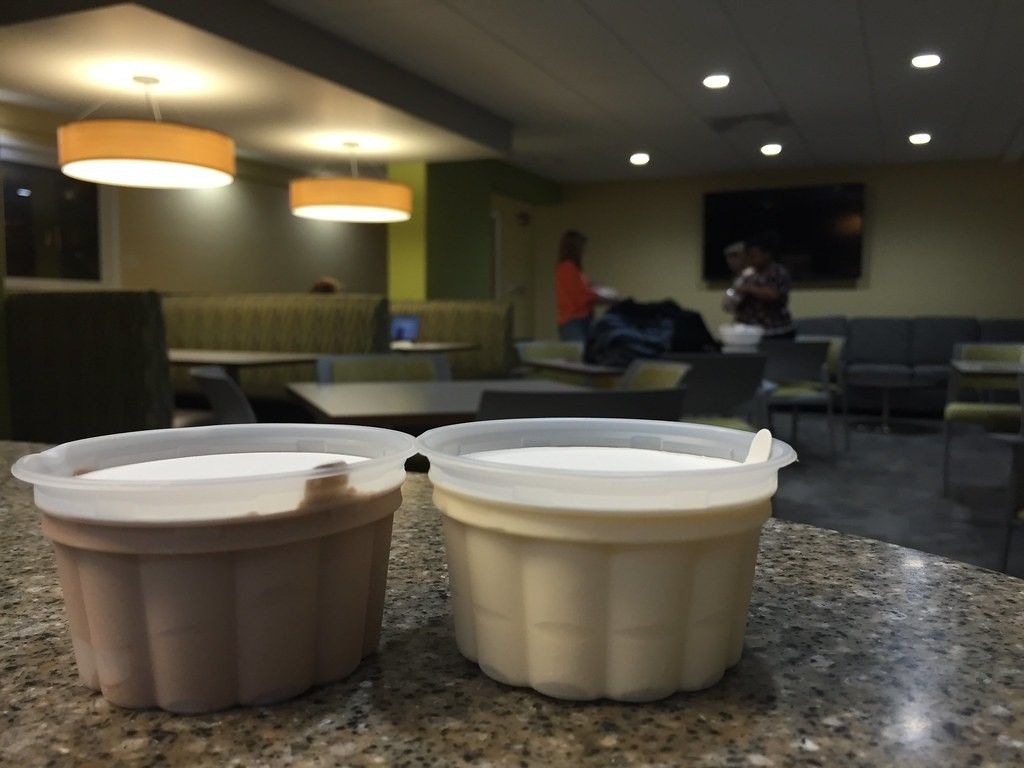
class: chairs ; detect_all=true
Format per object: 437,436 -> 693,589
766,336 -> 854,460
942,343 -> 1024,493
798,313 -> 1024,434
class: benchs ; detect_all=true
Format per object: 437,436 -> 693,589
158,297 -> 511,422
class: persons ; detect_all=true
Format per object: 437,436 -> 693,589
556,229 -> 633,342
721,243 -> 798,342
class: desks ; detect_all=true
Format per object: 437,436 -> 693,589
0,440 -> 1024,768
166,349 -> 321,418
527,357 -> 626,386
285,380 -> 600,434
389,341 -> 483,378
950,360 -> 1024,526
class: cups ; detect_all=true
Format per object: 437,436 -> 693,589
415,416 -> 798,702
11,422 -> 415,717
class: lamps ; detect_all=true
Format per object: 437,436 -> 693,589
289,140 -> 414,223
57,75 -> 236,190
